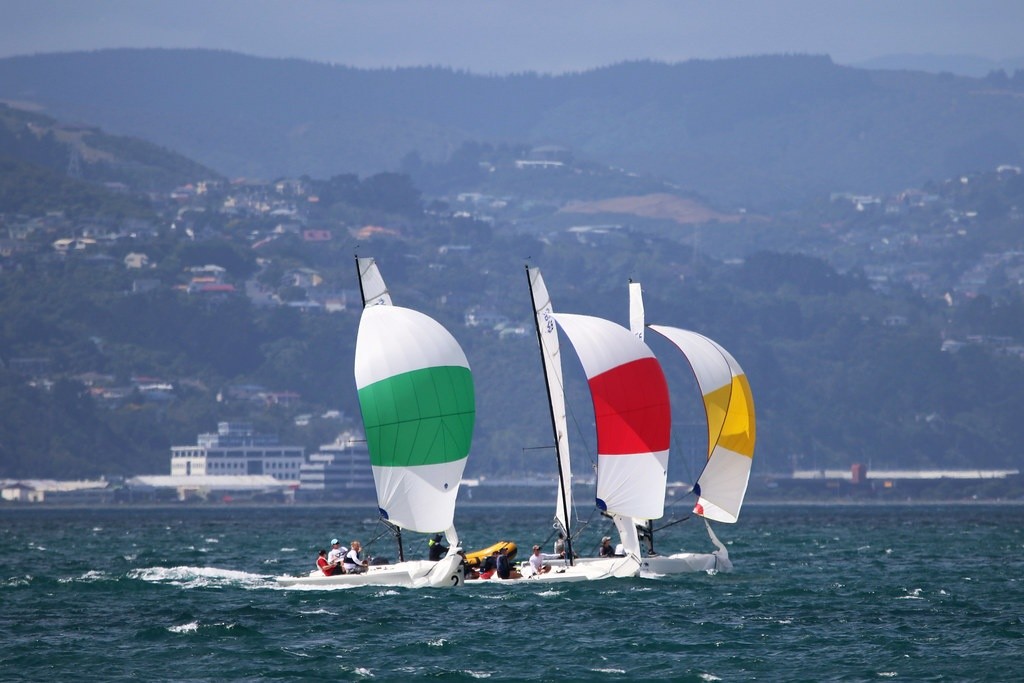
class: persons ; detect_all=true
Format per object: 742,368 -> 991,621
553,532 -> 576,558
345,542 -> 369,573
529,544 -> 566,576
497,548 -> 523,578
599,536 -> 616,558
428,533 -> 450,560
327,538 -> 349,564
317,549 -> 346,575
479,551 -> 501,579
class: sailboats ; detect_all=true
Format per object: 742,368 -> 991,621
540,278 -> 757,575
465,265 -> 672,587
275,253 -> 477,588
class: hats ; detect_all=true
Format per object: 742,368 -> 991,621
330,539 -> 340,545
602,536 -> 612,543
318,548 -> 326,555
498,547 -> 508,553
533,544 -> 543,550
